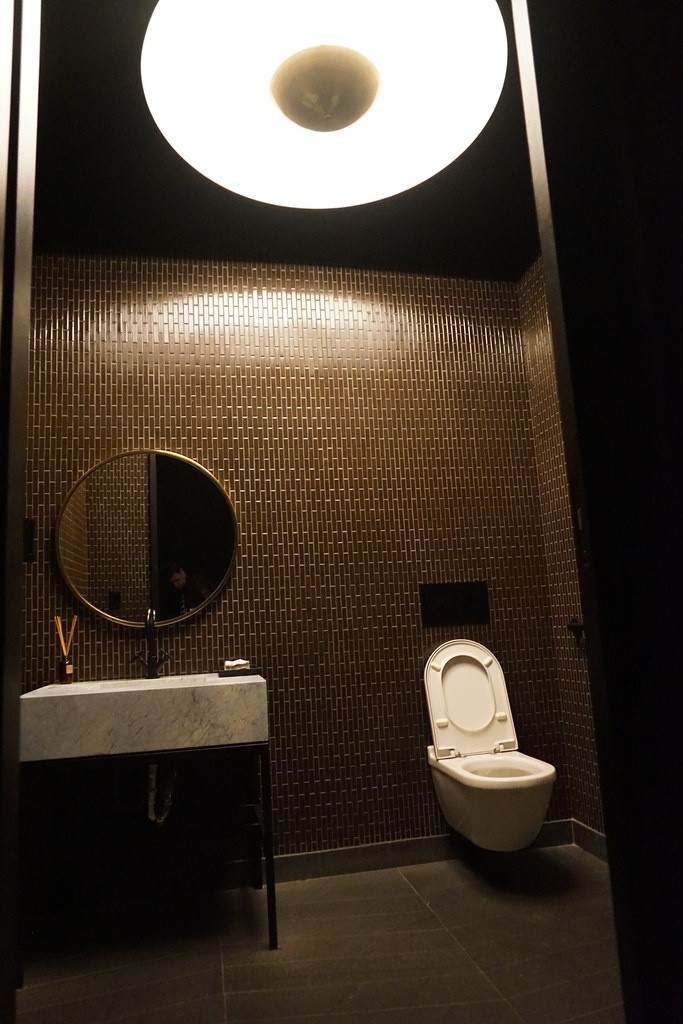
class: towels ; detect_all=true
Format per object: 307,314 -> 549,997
224,658 -> 251,670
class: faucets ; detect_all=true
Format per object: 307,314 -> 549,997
130,607 -> 175,678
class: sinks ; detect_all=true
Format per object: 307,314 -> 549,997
20,673 -> 268,762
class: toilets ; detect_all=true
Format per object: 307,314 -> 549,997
423,638 -> 557,853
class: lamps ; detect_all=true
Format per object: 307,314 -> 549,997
140,0 -> 514,212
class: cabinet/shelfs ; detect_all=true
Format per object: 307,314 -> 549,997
12,743 -> 277,989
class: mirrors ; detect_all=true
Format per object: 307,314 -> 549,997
53,447 -> 240,631
